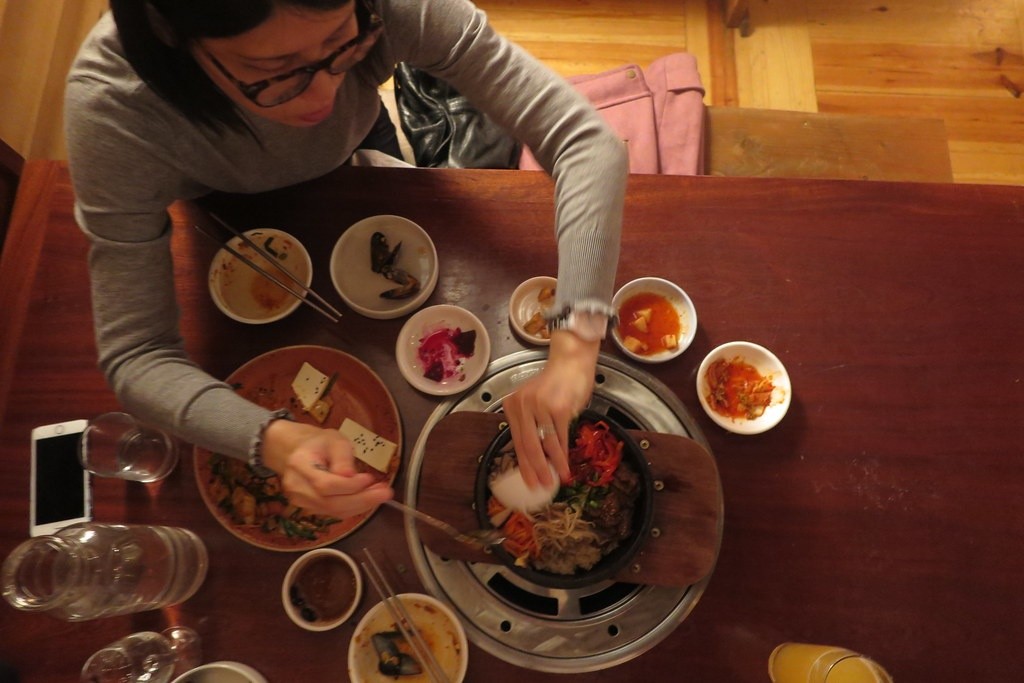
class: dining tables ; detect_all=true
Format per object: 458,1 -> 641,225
0,156 -> 1024,683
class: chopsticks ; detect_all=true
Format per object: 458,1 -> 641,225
361,548 -> 449,683
195,212 -> 342,323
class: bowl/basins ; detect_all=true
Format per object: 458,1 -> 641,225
332,216 -> 438,319
172,662 -> 264,683
475,410 -> 653,590
282,547 -> 360,632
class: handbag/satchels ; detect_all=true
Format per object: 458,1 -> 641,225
393,62 -> 524,169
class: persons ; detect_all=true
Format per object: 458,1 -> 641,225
64,1 -> 628,522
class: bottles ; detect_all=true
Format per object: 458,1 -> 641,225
4,522 -> 207,624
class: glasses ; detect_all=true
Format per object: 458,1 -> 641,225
195,0 -> 387,108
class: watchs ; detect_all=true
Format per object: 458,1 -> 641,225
545,304 -> 611,342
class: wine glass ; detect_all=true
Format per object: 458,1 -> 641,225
82,627 -> 202,683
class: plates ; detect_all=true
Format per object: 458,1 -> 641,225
509,277 -> 559,346
348,593 -> 467,683
195,346 -> 402,552
697,341 -> 792,435
397,306 -> 490,396
209,230 -> 312,324
611,277 -> 697,362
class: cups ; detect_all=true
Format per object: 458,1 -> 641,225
78,413 -> 178,484
768,642 -> 892,683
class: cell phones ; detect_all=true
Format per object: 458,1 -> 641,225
29,421 -> 92,537
